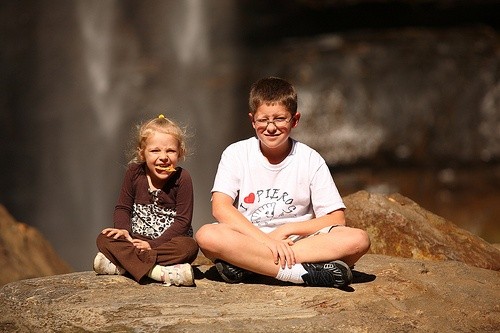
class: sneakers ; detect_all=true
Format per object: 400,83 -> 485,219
300,260 -> 353,288
163,263 -> 195,287
215,259 -> 257,283
93,251 -> 126,275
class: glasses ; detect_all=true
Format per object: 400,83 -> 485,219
251,115 -> 294,128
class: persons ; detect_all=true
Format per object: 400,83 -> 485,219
94,115 -> 198,286
195,76 -> 371,288
